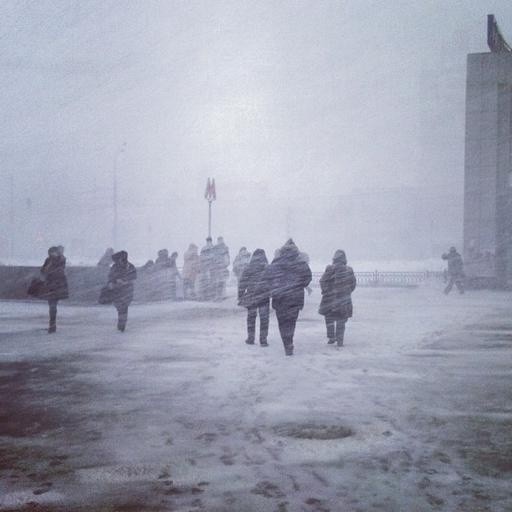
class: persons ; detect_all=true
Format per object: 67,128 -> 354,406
40,246 -> 69,332
258,237 -> 313,356
106,249 -> 138,334
95,248 -> 116,266
138,236 -> 314,305
441,247 -> 466,295
316,249 -> 357,348
237,248 -> 272,347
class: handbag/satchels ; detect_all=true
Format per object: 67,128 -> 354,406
27,277 -> 45,296
98,286 -> 112,304
237,292 -> 255,307
